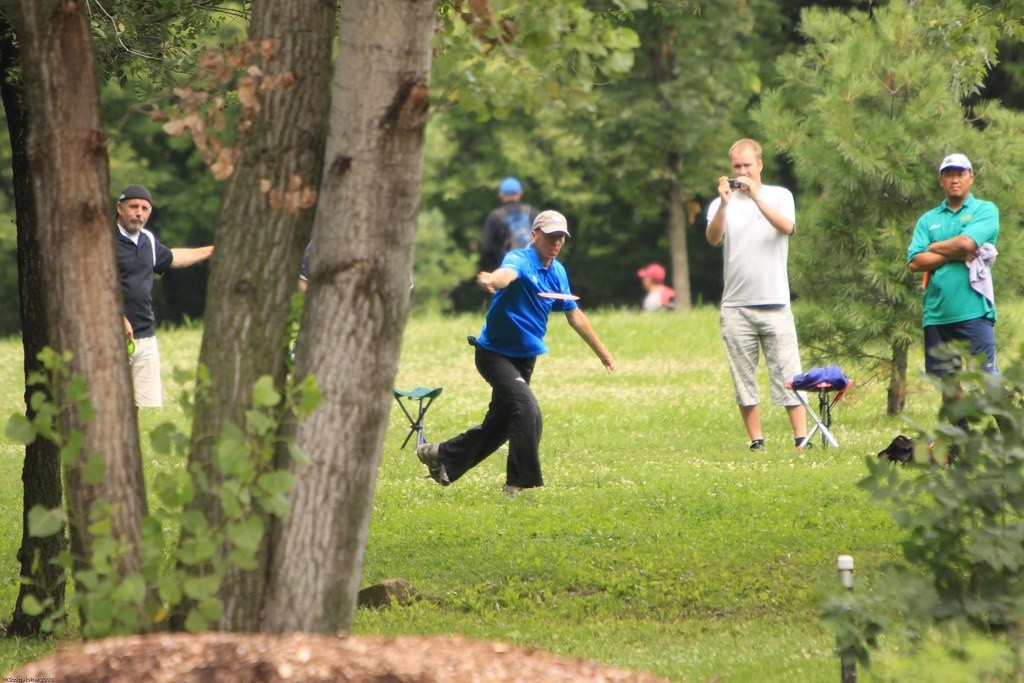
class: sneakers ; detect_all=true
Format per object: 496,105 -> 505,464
417,443 -> 448,485
502,485 -> 521,496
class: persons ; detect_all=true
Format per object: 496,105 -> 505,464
297,241 -> 414,292
636,262 -> 676,313
480,177 -> 542,266
417,209 -> 613,497
114,186 -> 215,408
706,138 -> 813,453
907,154 -> 1017,435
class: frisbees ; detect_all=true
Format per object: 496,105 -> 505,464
536,292 -> 581,300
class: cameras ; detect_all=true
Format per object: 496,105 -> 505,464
729,178 -> 742,188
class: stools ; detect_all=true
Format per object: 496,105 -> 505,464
784,379 -> 851,449
393,385 -> 444,451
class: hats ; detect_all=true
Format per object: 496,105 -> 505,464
115,185 -> 151,219
532,209 -> 570,237
500,176 -> 521,194
637,264 -> 666,283
939,153 -> 973,174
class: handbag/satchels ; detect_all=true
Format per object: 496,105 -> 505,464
877,436 -> 952,466
